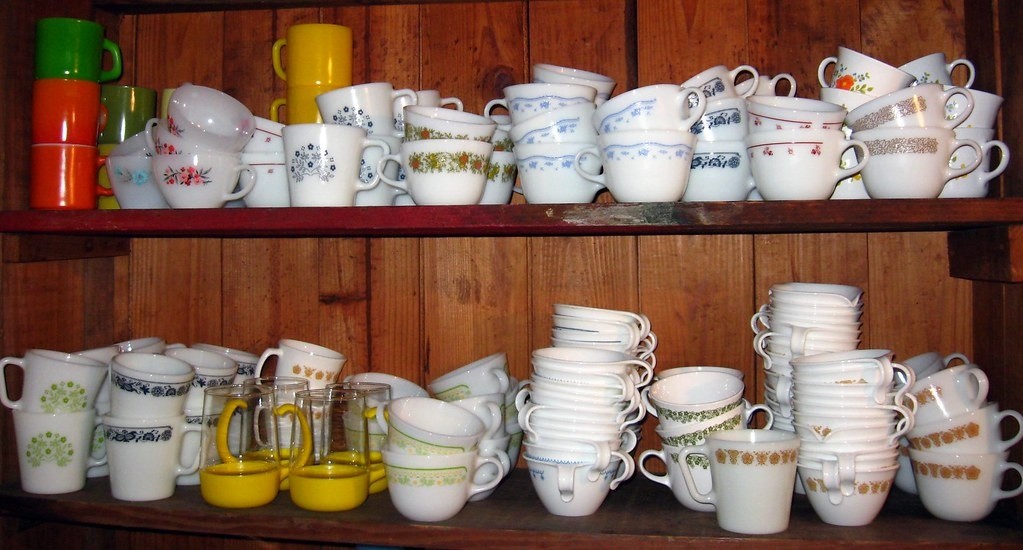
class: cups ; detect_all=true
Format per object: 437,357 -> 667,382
752,282 -> 917,526
100,47 -> 1011,211
30,16 -> 117,209
638,364 -> 799,534
0,335 -> 524,523
892,351 -> 1023,522
272,23 -> 352,88
513,304 -> 658,517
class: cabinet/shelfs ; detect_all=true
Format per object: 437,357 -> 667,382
0,0 -> 1023,550
